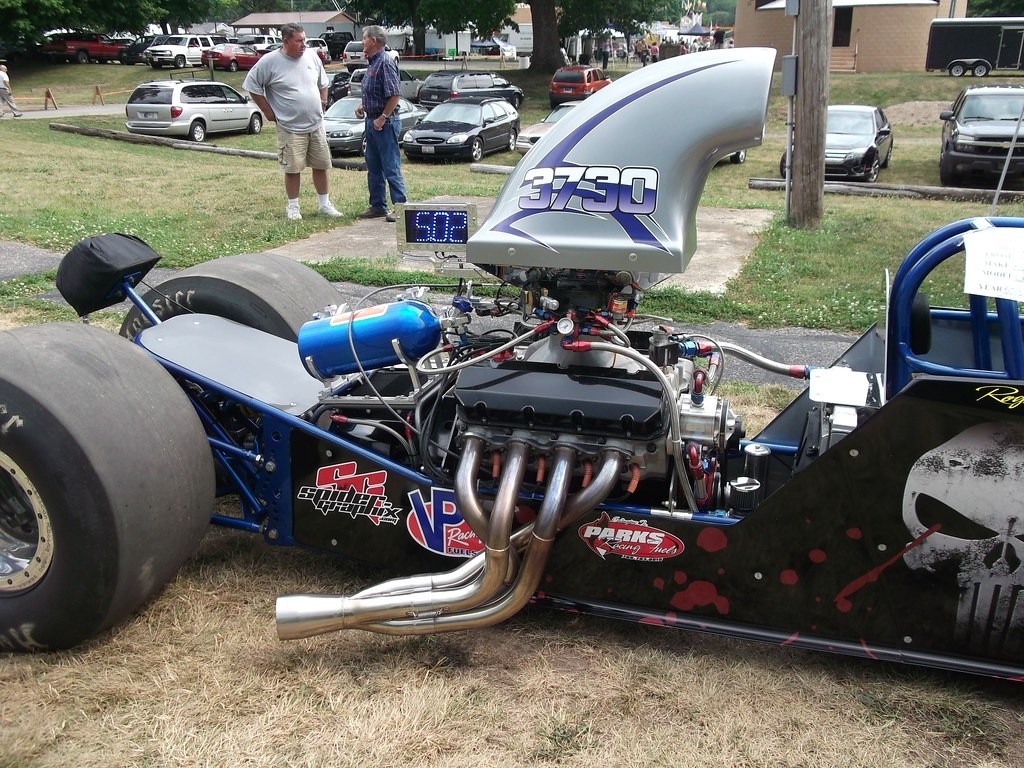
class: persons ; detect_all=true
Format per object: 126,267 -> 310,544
355,27 -> 407,221
650,42 -> 659,63
0,64 -> 23,117
661,37 -> 705,55
635,40 -> 650,67
713,26 -> 725,49
192,39 -> 198,47
602,39 -> 610,69
242,23 -> 344,220
317,44 -> 325,66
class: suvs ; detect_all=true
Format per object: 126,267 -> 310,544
549,65 -> 612,110
236,34 -> 282,51
418,69 -> 525,110
125,78 -> 264,144
938,86 -> 1024,186
343,40 -> 400,73
318,32 -> 355,61
142,35 -> 230,69
348,68 -> 425,103
303,37 -> 333,66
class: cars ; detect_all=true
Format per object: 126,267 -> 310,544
780,105 -> 894,183
201,43 -> 263,72
515,100 -> 584,157
266,43 -> 283,51
403,95 -> 521,163
323,95 -> 430,156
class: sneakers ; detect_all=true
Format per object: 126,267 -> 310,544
318,202 -> 343,218
286,206 -> 302,220
386,211 -> 395,222
359,208 -> 388,218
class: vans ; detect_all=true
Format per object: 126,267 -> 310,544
120,34 -> 171,65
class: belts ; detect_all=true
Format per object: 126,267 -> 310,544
367,110 -> 398,118
0,88 -> 6,89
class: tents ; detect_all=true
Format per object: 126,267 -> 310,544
677,23 -> 710,38
471,36 -> 498,47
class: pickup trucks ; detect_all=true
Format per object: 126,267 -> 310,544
40,32 -> 130,65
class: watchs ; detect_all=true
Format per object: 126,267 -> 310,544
321,100 -> 328,106
382,114 -> 388,120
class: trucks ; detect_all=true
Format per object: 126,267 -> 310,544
925,16 -> 1024,78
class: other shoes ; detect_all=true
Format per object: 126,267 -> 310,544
14,113 -> 22,117
0,113 -> 4,117
